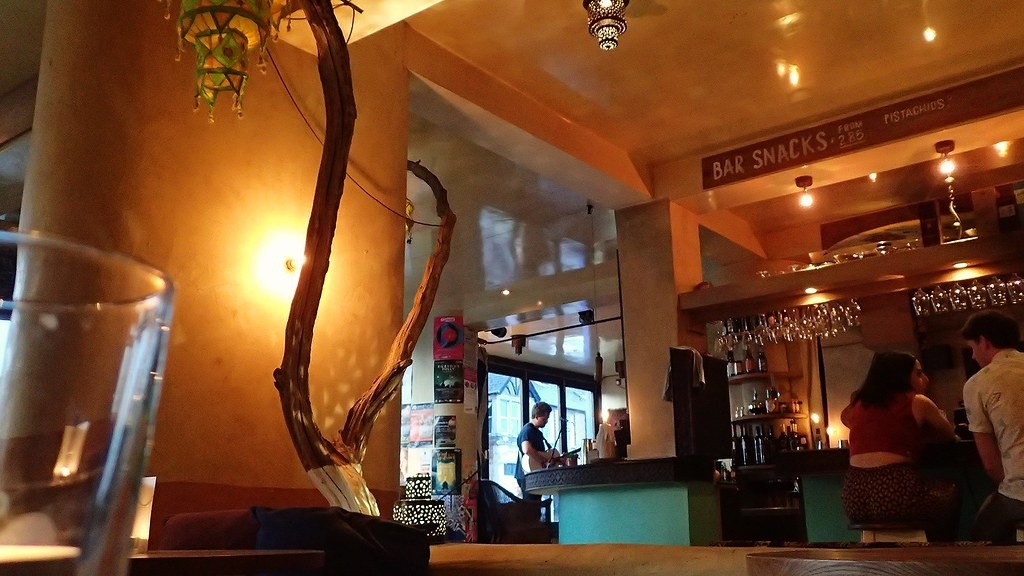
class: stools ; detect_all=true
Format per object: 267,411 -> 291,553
848,523 -> 944,543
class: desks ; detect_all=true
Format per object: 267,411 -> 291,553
128,549 -> 326,576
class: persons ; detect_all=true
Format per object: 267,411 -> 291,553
960,309 -> 1024,543
513,402 -> 567,508
841,348 -> 959,525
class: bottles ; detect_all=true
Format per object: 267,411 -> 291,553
744,345 -> 756,372
731,419 -> 809,465
758,345 -> 767,372
815,428 -> 822,449
727,347 -> 735,377
735,386 -> 803,418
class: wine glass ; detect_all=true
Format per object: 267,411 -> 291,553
715,299 -> 862,352
912,273 -> 1024,316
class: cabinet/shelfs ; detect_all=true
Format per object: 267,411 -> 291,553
729,371 -> 805,475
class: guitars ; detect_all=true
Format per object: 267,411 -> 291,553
521,448 -> 582,475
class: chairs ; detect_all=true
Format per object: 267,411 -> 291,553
480,479 -> 552,544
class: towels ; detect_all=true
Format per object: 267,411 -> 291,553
660,344 -> 706,402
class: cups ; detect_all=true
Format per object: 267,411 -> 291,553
0,223 -> 174,576
